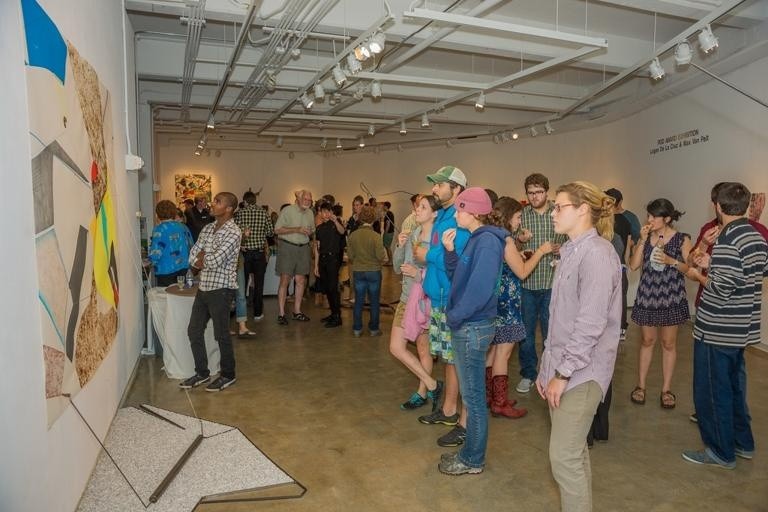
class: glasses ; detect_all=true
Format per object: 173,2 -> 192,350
526,189 -> 546,197
550,202 -> 577,213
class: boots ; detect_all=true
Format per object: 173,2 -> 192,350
490,375 -> 528,418
486,366 -> 517,408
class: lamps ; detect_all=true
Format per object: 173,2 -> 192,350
275,33 -> 555,159
646,10 -> 665,80
195,115 -> 214,156
672,23 -> 719,67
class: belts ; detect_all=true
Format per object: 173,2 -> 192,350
278,238 -> 309,247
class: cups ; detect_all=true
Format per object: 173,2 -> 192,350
523,249 -> 533,261
410,234 -> 422,248
176,276 -> 185,288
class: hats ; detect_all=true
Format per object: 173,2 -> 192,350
454,186 -> 493,215
424,165 -> 467,189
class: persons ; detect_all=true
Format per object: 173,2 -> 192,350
179,191 -> 242,391
176,208 -> 202,244
347,206 -> 388,338
314,202 -> 347,328
606,188 -> 642,340
629,198 -> 693,408
681,181 -> 766,469
485,197 -> 554,419
192,192 -> 215,226
687,183 -> 766,422
402,194 -> 424,234
233,192 -> 274,320
438,187 -> 511,475
346,195 -> 363,235
369,198 -> 380,234
262,204 -> 291,254
389,196 -> 444,413
149,200 -> 194,287
274,190 -> 315,324
382,202 -> 394,266
413,167 -> 470,446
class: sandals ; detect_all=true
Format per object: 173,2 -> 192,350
631,386 -> 646,405
660,391 -> 675,409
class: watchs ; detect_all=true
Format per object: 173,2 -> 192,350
673,260 -> 678,268
555,368 -> 570,381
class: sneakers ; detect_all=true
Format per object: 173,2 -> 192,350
254,312 -> 264,320
178,374 -> 211,389
619,328 -> 626,341
418,408 -> 460,426
312,296 -> 381,337
515,378 -> 535,393
204,375 -> 237,392
681,413 -> 754,470
437,422 -> 486,475
427,380 -> 445,414
278,314 -> 288,325
400,392 -> 427,410
292,312 -> 310,321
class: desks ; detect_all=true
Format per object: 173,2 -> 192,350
247,254 -> 294,297
147,282 -> 224,379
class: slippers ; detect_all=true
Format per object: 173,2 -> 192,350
238,329 -> 257,338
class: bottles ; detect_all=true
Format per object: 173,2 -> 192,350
269,245 -> 276,256
185,269 -> 194,289
654,235 -> 664,253
306,224 -> 312,235
